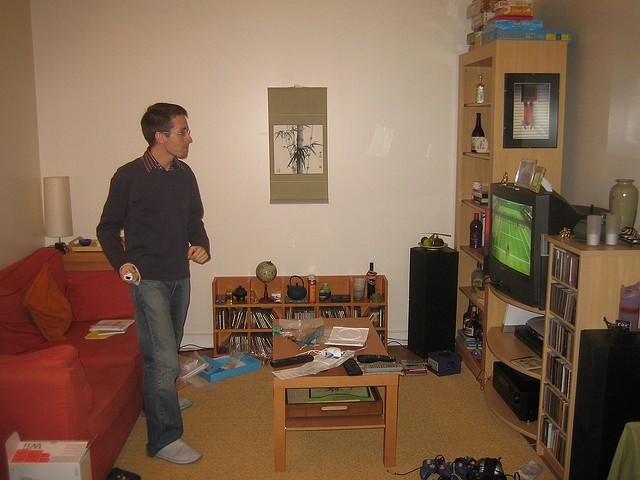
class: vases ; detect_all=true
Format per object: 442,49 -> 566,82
609,177 -> 640,237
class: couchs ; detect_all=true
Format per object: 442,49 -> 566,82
1,247 -> 145,479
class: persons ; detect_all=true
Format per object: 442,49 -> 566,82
96,101 -> 212,464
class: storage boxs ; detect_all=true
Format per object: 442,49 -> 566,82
4,430 -> 100,480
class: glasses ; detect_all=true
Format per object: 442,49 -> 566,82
162,130 -> 191,136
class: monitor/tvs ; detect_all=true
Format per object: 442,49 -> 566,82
486,181 -> 608,311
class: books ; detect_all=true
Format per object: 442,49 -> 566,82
546,354 -> 572,400
551,284 -> 576,324
541,415 -> 567,466
544,386 -> 568,432
548,319 -> 574,362
552,247 -> 579,288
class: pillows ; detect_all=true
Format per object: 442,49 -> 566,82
64,269 -> 137,322
21,260 -> 74,342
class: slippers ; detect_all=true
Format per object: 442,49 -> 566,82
155,439 -> 202,464
141,397 -> 192,416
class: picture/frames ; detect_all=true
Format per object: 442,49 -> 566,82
266,86 -> 328,205
502,73 -> 561,150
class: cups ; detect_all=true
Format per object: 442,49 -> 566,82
606,212 -> 620,246
587,214 -> 603,248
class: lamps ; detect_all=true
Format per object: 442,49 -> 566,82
42,175 -> 74,255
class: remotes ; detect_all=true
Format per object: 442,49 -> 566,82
344,357 -> 363,375
360,354 -> 397,363
364,362 -> 402,371
270,354 -> 315,369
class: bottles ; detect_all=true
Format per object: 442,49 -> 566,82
308,275 -> 316,303
471,260 -> 485,291
320,283 -> 331,300
475,75 -> 485,104
464,307 -> 481,338
469,213 -> 485,249
462,299 -> 473,332
365,262 -> 377,299
471,113 -> 485,153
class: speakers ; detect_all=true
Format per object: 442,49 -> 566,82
569,327 -> 640,480
407,245 -> 458,358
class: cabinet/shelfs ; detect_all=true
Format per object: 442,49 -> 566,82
481,284 -> 546,441
535,233 -> 640,480
452,39 -> 569,384
211,274 -> 388,358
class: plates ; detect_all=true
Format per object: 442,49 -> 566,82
418,241 -> 448,252
